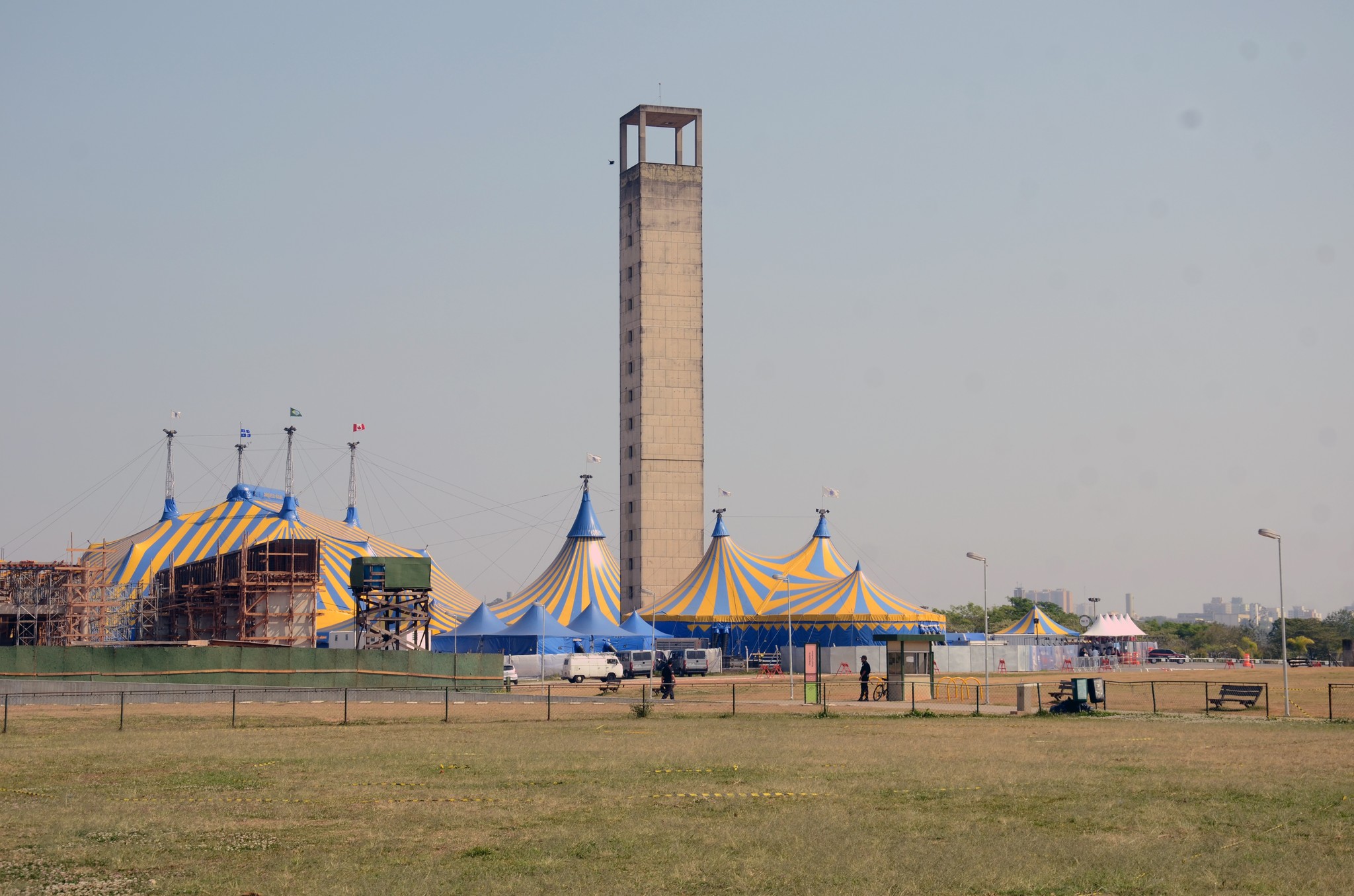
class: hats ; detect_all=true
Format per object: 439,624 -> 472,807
860,655 -> 867,661
660,687 -> 665,693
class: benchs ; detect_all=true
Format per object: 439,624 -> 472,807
1208,682 -> 1263,710
1047,679 -> 1074,701
652,681 -> 675,696
598,678 -> 622,694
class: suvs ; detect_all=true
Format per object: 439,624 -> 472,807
1148,649 -> 1190,664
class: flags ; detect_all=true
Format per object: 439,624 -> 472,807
290,407 -> 302,417
353,424 -> 365,432
822,486 -> 839,498
171,410 -> 181,419
587,453 -> 601,464
241,429 -> 251,437
719,488 -> 731,498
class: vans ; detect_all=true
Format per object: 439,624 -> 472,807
612,649 -> 654,680
654,648 -> 709,677
560,652 -> 623,684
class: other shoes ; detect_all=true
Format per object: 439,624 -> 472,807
863,698 -> 869,701
857,698 -> 863,702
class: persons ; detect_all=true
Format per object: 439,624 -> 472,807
1079,645 -> 1122,666
660,659 -> 677,700
857,655 -> 871,701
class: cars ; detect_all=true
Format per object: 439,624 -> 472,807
503,664 -> 518,685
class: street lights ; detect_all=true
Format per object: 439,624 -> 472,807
772,574 -> 794,701
445,611 -> 457,654
640,588 -> 656,699
531,601 -> 546,696
1088,597 -> 1101,622
966,552 -> 990,706
1258,527 -> 1289,716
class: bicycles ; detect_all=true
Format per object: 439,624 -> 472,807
872,678 -> 887,701
